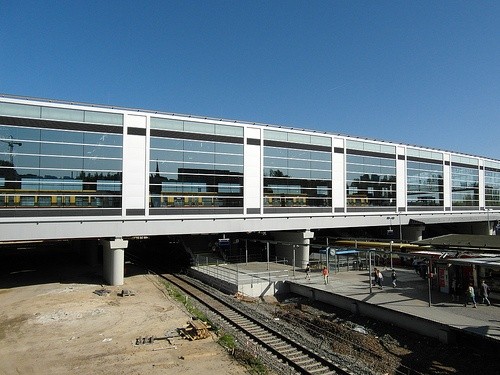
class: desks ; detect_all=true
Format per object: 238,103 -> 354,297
187,319 -> 209,339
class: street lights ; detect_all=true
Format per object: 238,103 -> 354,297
368,248 -> 377,295
386,216 -> 395,230
389,241 -> 394,269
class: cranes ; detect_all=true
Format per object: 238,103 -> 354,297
0,135 -> 22,165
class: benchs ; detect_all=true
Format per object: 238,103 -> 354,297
177,328 -> 191,339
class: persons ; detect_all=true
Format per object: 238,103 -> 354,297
305,264 -> 311,280
352,260 -> 368,270
372,267 -> 378,286
479,280 -> 491,306
377,270 -> 384,290
371,254 -> 389,266
463,283 -> 477,308
412,258 -> 419,275
391,269 -> 397,288
321,266 -> 328,285
